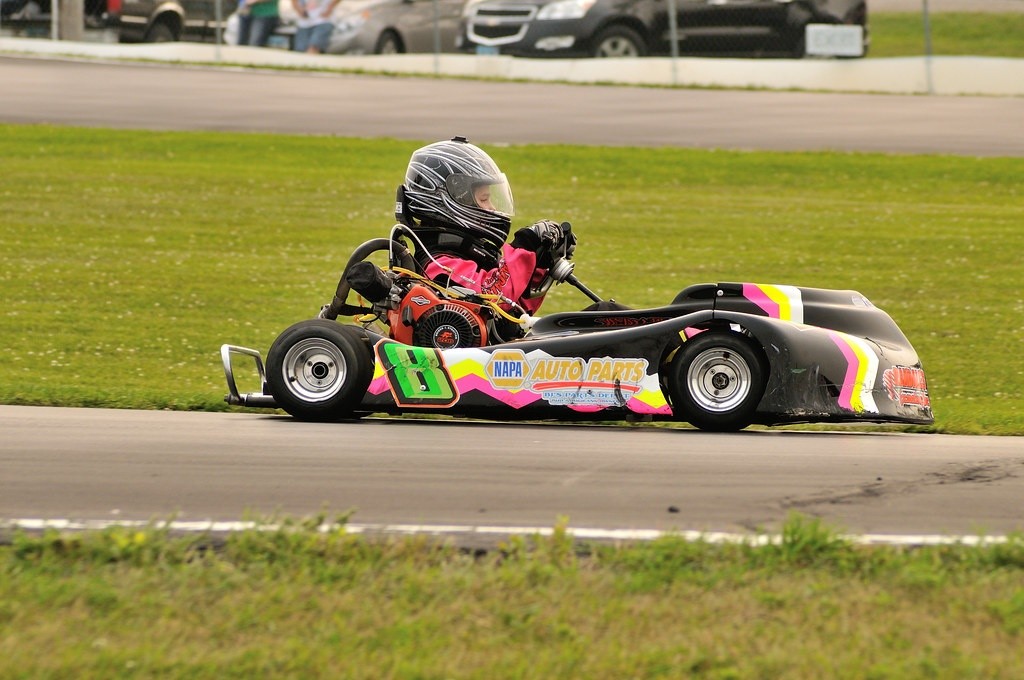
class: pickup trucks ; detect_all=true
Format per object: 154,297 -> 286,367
1,0 -> 239,43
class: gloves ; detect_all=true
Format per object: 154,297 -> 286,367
514,218 -> 575,268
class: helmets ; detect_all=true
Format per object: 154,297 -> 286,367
396,136 -> 514,265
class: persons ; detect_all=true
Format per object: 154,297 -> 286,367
238,0 -> 280,46
291,0 -> 340,54
402,137 -> 574,343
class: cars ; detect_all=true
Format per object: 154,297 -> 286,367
456,0 -> 869,59
267,0 -> 466,56
